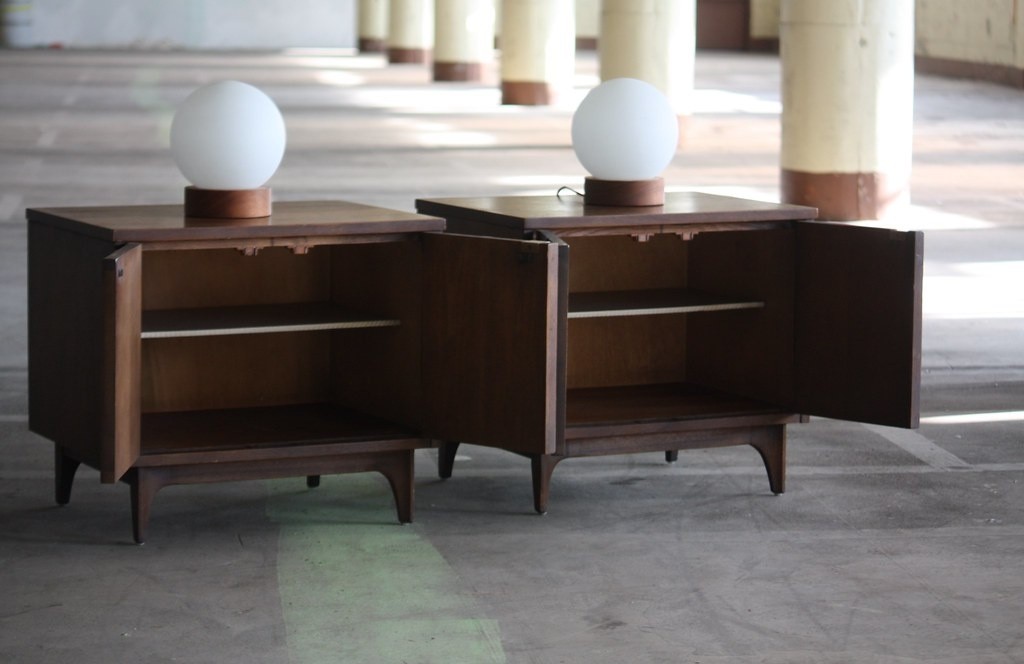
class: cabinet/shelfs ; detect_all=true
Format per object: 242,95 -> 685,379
22,201 -> 560,550
418,192 -> 924,516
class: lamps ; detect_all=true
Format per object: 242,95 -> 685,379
557,78 -> 679,207
168,80 -> 287,218
